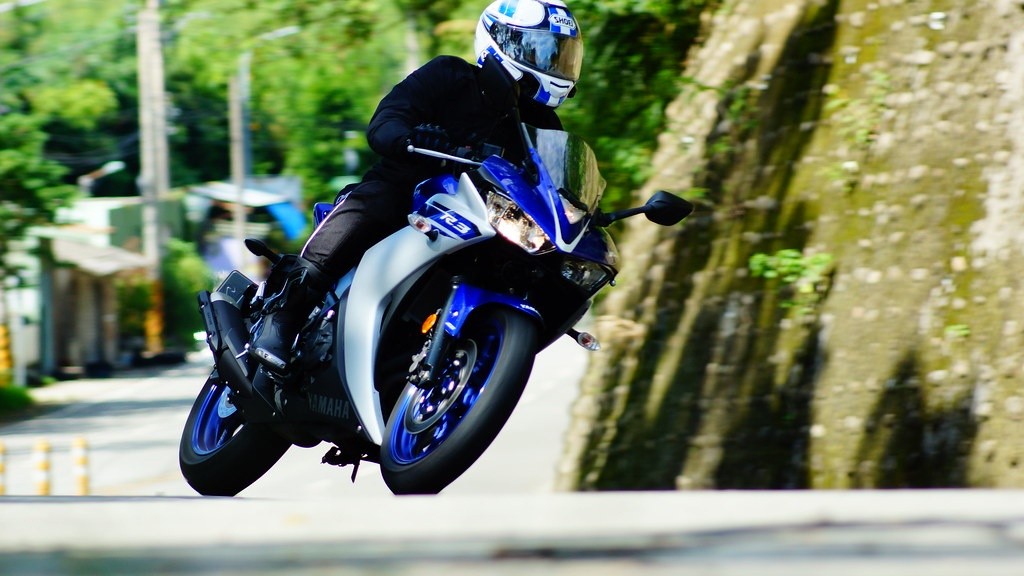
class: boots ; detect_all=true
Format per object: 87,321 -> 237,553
248,257 -> 328,373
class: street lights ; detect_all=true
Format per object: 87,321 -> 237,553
225,22 -> 303,192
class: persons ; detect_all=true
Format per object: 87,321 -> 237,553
250,0 -> 583,372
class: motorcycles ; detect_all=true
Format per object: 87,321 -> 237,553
176,48 -> 694,500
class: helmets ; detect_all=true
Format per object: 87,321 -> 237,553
474,0 -> 583,108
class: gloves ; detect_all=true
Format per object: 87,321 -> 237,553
410,122 -> 451,154
